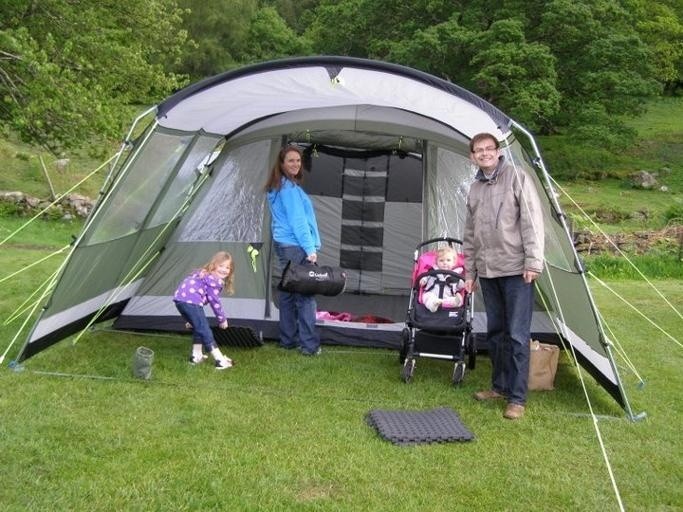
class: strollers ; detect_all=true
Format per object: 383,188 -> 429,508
398,237 -> 478,387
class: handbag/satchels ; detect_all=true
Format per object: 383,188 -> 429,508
277,255 -> 349,298
528,339 -> 560,392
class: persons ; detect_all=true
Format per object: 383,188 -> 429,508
262,144 -> 328,358
419,246 -> 465,313
461,133 -> 546,418
170,250 -> 239,370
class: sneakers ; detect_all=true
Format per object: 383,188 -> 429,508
215,354 -> 237,370
505,402 -> 524,420
188,353 -> 208,364
474,390 -> 502,402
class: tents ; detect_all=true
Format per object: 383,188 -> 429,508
13,54 -> 638,426
